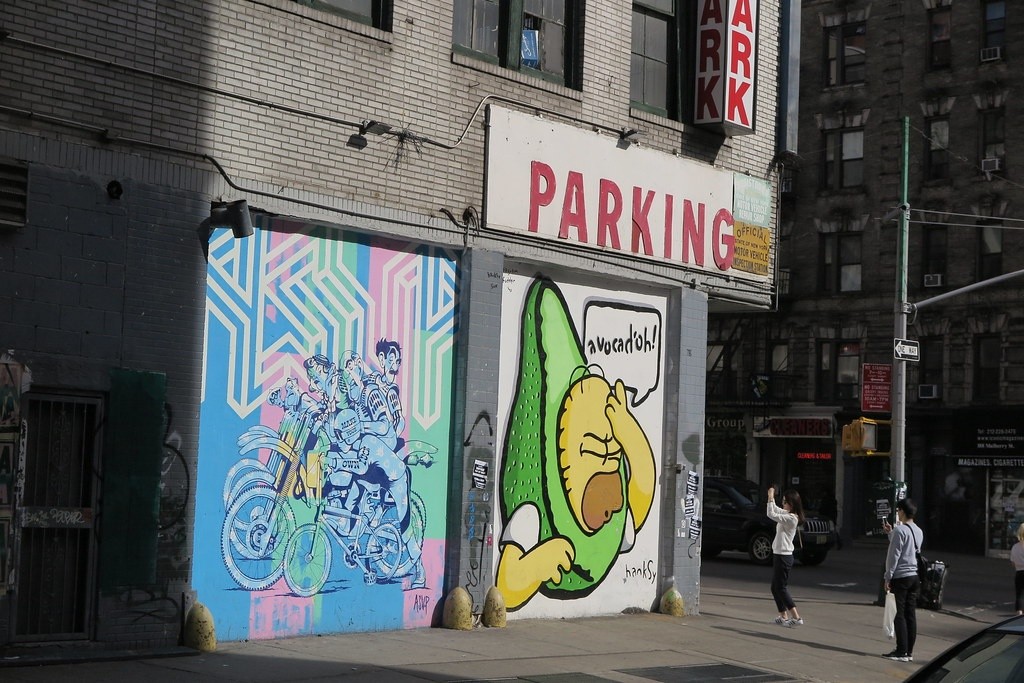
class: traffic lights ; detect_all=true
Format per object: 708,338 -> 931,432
842,421 -> 878,454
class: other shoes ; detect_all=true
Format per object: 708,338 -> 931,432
1016,610 -> 1023,616
907,652 -> 913,660
881,648 -> 909,661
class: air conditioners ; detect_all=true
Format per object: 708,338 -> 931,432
918,384 -> 942,399
925,274 -> 944,288
980,46 -> 1001,63
982,157 -> 1004,172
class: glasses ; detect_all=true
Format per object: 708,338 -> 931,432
782,499 -> 789,505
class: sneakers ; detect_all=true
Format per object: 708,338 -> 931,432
772,616 -> 788,625
785,617 -> 804,627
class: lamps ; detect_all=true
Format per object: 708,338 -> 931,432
229,199 -> 255,238
624,127 -> 646,140
362,118 -> 391,135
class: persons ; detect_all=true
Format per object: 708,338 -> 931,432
766,487 -> 803,628
1011,522 -> 1024,617
881,499 -> 924,664
819,488 -> 843,549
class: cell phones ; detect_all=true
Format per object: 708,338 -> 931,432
882,516 -> 887,527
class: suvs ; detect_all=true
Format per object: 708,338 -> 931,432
702,474 -> 838,567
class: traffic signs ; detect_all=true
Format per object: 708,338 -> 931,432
894,338 -> 920,361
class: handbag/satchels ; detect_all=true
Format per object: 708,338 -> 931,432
882,589 -> 896,639
916,552 -> 928,583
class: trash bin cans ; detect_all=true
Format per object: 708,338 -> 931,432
915,560 -> 949,609
